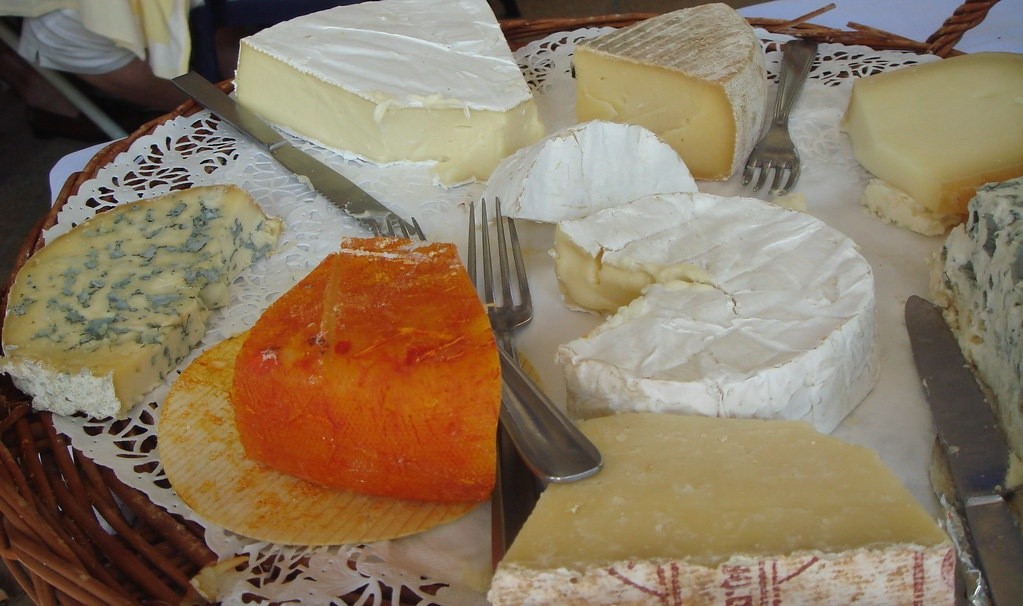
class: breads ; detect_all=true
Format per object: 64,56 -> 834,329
0,0 -> 1023,604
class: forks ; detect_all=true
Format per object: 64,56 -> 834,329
464,197 -> 535,560
370,217 -> 604,483
742,40 -> 818,198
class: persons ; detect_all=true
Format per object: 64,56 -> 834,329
0,0 -> 360,141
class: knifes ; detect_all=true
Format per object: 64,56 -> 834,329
170,71 -> 416,243
904,294 -> 1023,606
